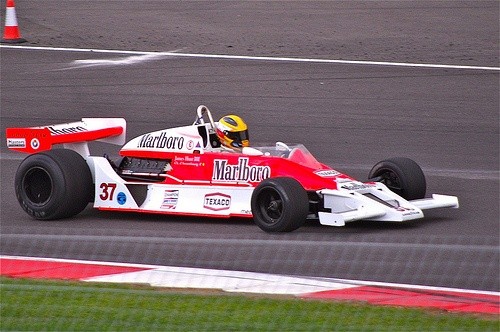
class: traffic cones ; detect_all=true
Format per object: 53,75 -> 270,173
1,0 -> 25,44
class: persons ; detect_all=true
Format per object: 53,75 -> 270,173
216,114 -> 250,154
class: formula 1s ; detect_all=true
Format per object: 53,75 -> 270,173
3,104 -> 460,232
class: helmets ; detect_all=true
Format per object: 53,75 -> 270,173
217,116 -> 250,151
